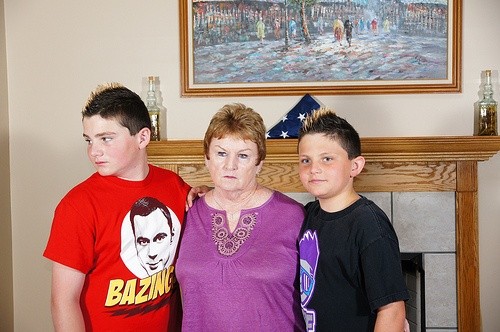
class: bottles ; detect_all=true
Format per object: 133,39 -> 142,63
145,75 -> 161,142
478,69 -> 497,136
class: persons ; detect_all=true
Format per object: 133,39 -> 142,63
175,102 -> 410,332
130,197 -> 174,276
185,108 -> 410,332
42,82 -> 193,332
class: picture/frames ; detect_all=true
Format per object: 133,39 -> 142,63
179,0 -> 462,96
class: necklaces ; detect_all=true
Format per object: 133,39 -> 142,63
212,182 -> 258,219
